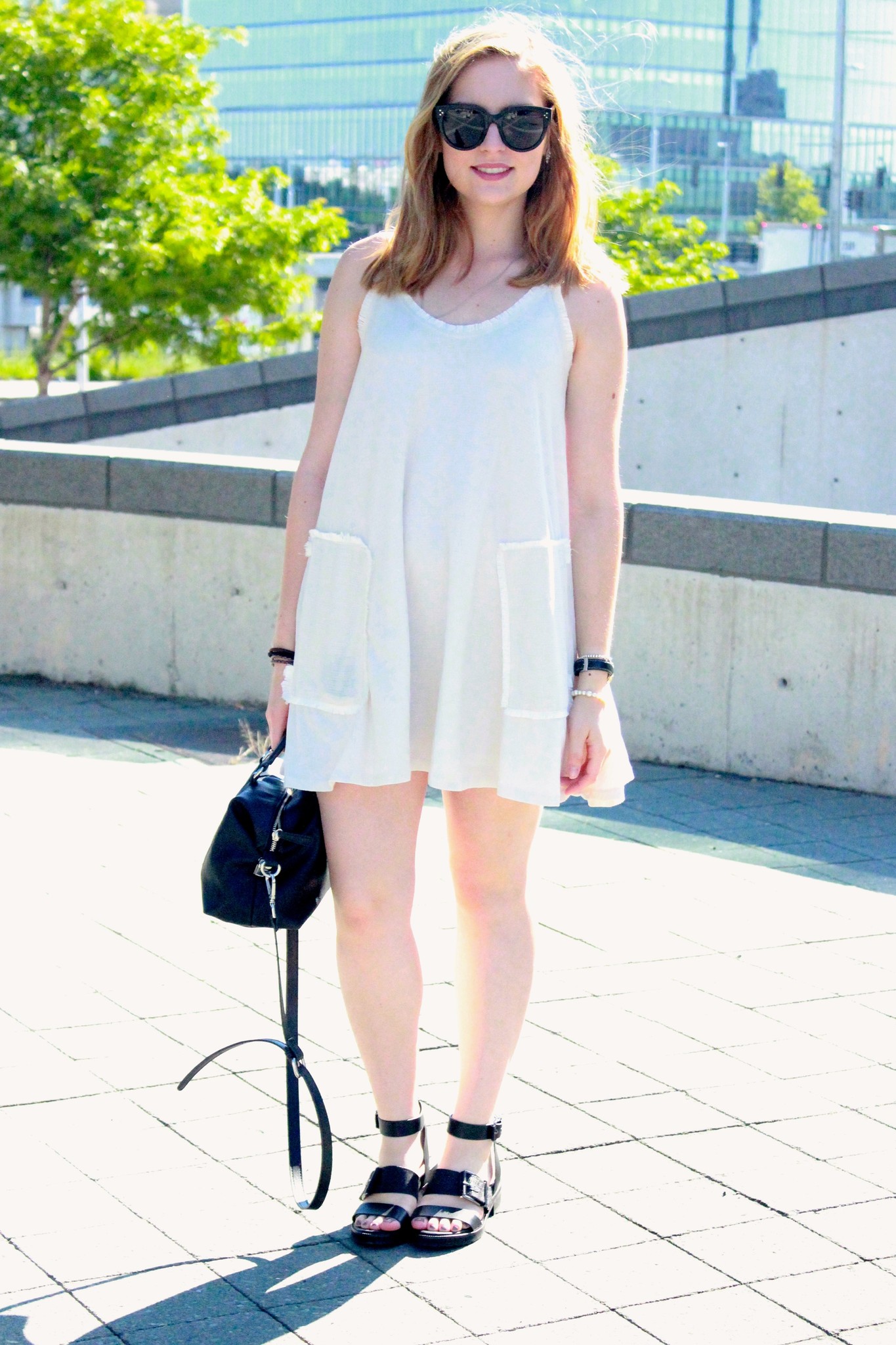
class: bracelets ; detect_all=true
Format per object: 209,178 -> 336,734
267,647 -> 296,667
570,653 -> 616,708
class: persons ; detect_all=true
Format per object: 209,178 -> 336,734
252,23 -> 639,1253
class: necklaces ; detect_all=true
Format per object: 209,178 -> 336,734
413,247 -> 529,320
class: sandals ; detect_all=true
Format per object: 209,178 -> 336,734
351,1098 -> 431,1246
409,1113 -> 504,1249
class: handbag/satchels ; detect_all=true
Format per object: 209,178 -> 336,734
200,729 -> 331,933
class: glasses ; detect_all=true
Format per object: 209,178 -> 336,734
431,102 -> 556,153
